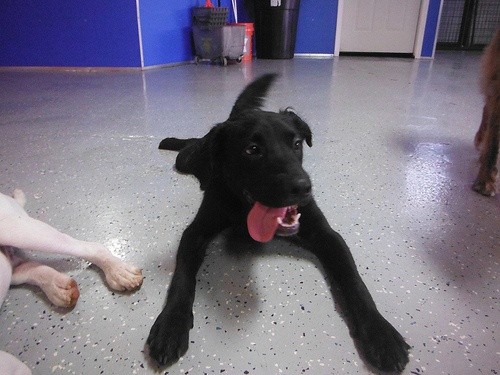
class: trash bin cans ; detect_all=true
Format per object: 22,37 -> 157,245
249,0 -> 300,58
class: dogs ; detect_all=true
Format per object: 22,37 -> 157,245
146,73 -> 413,375
469,28 -> 500,198
0,186 -> 144,375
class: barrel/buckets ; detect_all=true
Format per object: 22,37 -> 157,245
227,22 -> 254,63
